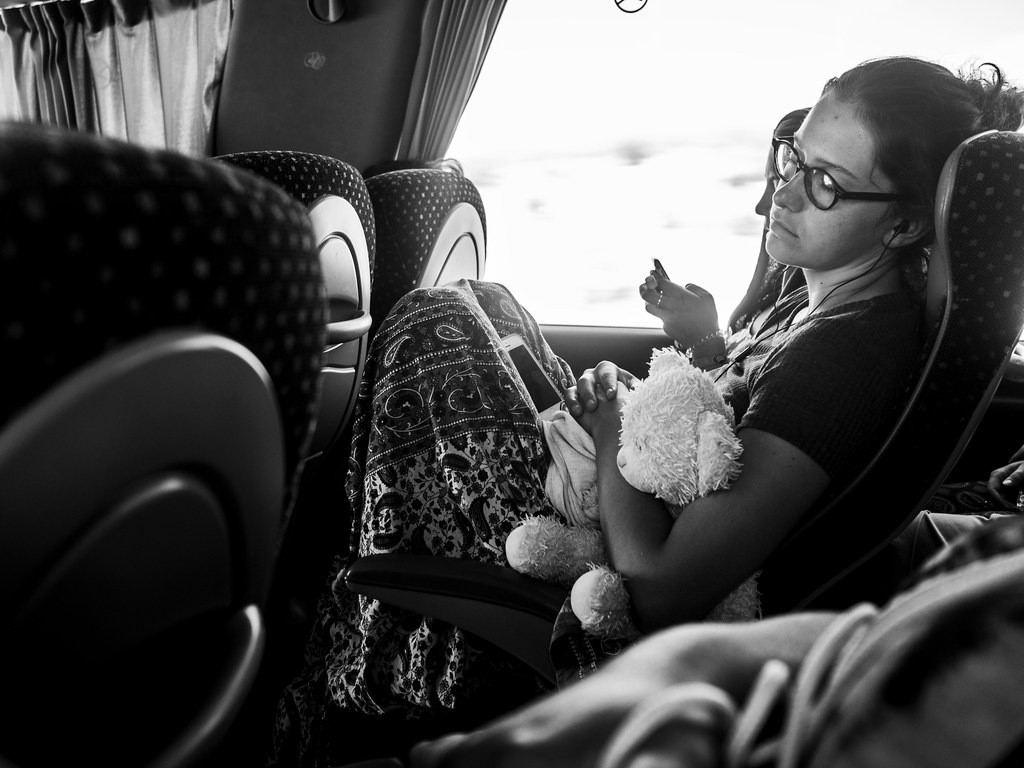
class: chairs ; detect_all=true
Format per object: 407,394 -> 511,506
1,109 -> 1024,767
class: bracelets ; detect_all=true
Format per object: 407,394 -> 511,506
692,330 -> 725,353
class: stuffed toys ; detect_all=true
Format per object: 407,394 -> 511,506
505,347 -> 743,642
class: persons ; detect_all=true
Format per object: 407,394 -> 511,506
273,57 -> 1024,768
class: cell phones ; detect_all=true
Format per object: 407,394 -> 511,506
652,257 -> 670,282
502,332 -> 563,421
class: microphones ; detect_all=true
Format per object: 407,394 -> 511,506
893,219 -> 909,237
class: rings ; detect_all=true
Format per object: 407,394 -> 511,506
656,294 -> 662,308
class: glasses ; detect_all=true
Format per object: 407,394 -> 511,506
769,132 -> 903,210
